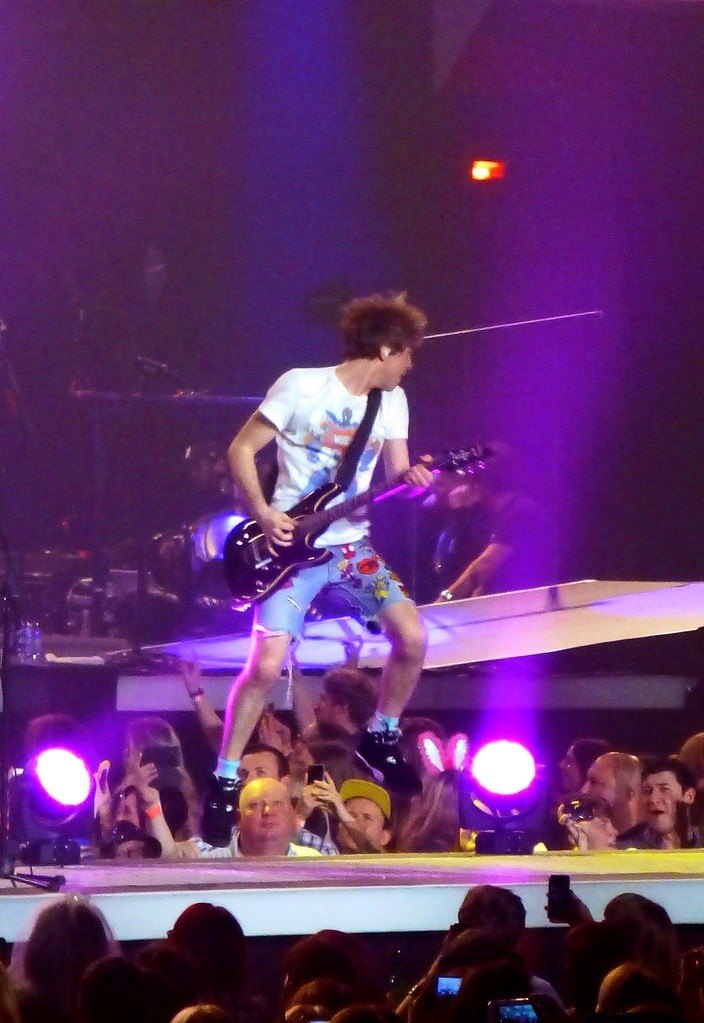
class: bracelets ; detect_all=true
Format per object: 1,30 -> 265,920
192,693 -> 206,702
441,590 -> 454,601
189,686 -> 205,699
407,984 -> 423,997
144,802 -> 162,819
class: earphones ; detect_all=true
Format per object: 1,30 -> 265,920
385,347 -> 392,357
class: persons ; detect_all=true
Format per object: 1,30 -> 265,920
198,292 -> 433,848
436,440 -> 560,605
0,632 -> 704,1023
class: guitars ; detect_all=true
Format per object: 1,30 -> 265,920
223,441 -> 500,607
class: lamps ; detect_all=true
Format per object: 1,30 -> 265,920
472,737 -> 548,854
0,746 -> 94,893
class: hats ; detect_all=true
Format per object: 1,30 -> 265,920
337,778 -> 392,823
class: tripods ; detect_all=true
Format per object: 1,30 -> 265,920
0,583 -> 64,893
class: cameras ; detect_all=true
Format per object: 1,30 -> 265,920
435,975 -> 463,999
488,997 -> 538,1023
562,799 -> 594,821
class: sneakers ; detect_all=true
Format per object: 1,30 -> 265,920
354,724 -> 424,794
200,772 -> 245,849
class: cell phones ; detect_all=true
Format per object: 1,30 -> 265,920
547,875 -> 571,923
308,764 -> 326,785
